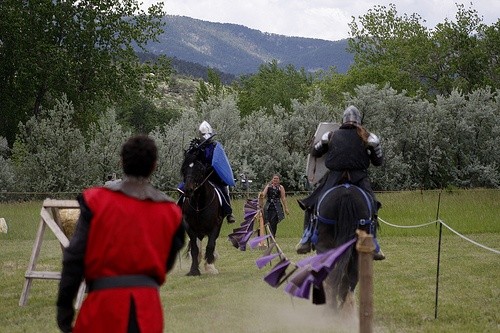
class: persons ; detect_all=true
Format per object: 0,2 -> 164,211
177,121 -> 235,223
57,135 -> 186,333
297,106 -> 385,260
262,176 -> 289,243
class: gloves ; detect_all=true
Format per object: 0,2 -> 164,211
56,304 -> 76,333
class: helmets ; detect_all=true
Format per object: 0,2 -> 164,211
342,105 -> 361,125
199,120 -> 213,138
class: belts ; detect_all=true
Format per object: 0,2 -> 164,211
85,273 -> 160,293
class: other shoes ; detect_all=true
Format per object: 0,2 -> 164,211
374,247 -> 385,261
226,215 -> 236,223
296,238 -> 313,253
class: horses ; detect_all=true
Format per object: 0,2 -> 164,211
175,145 -> 231,278
299,180 -> 379,329
